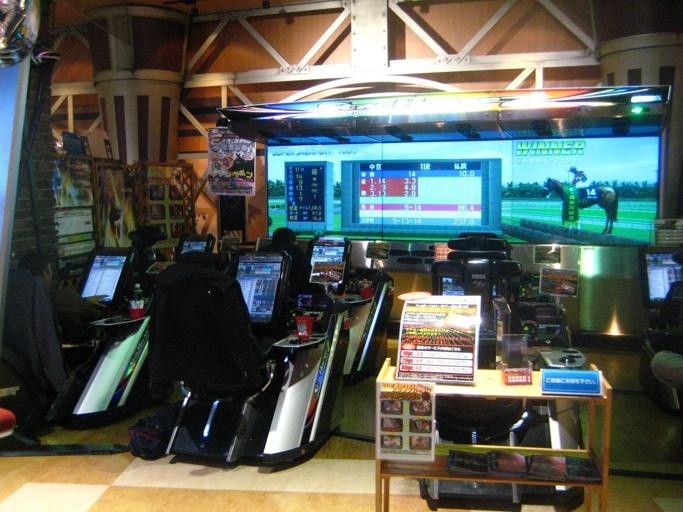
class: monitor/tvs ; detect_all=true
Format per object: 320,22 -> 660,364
310,237 -> 352,296
174,233 -> 215,262
232,250 -> 292,334
432,261 -> 511,296
77,247 -> 137,310
638,247 -> 683,309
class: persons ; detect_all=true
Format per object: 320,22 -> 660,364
252,223 -> 326,305
17,251 -> 109,377
569,164 -> 592,207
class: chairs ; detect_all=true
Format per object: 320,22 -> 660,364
431,232 -> 525,338
4,234 -> 327,466
638,296 -> 683,411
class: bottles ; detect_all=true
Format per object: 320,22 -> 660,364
129,283 -> 143,319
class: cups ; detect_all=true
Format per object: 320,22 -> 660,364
295,317 -> 313,343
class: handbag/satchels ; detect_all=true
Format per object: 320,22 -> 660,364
127,398 -> 184,460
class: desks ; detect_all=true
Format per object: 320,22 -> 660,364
373,358 -> 614,512
